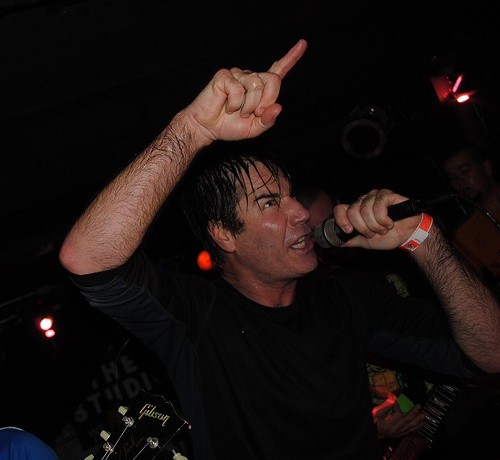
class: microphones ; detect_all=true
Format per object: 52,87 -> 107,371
315,196 -> 433,248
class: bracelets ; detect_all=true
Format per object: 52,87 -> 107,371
399,213 -> 434,254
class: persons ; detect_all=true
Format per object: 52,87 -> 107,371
59,39 -> 500,460
296,137 -> 500,443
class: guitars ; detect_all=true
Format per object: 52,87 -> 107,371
383,378 -> 457,460
84,393 -> 185,460
